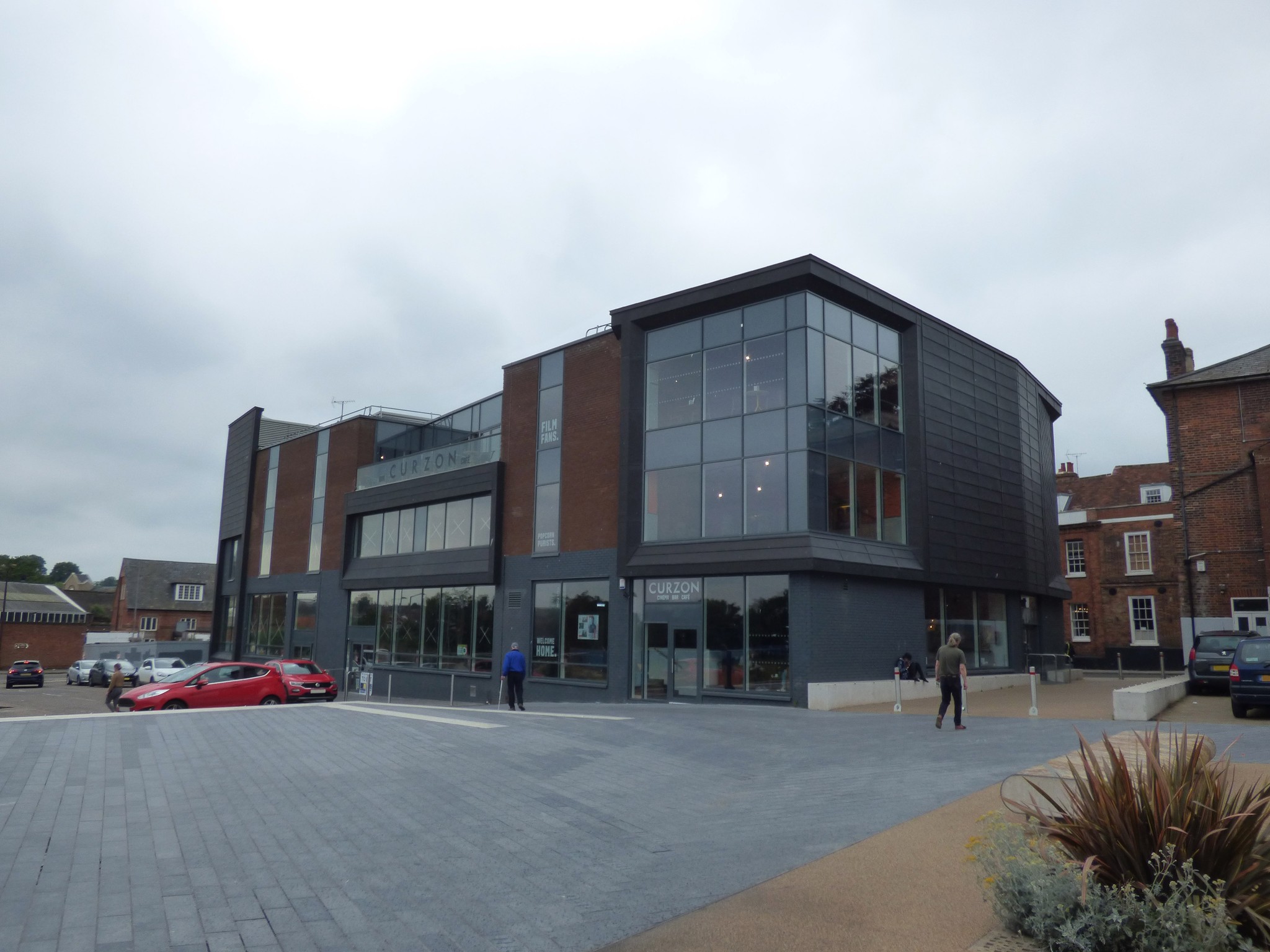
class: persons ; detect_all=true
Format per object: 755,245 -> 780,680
500,642 -> 525,711
898,652 -> 928,683
1065,639 -> 1075,664
934,633 -> 967,729
105,663 -> 125,712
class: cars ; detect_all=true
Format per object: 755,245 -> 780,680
115,662 -> 287,714
66,659 -> 98,686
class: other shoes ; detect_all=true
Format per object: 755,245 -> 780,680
955,724 -> 967,729
507,706 -> 516,711
935,716 -> 943,729
518,703 -> 525,711
912,676 -> 920,682
919,676 -> 929,682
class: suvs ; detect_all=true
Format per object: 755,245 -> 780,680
1228,636 -> 1270,719
265,658 -> 338,702
88,658 -> 137,688
134,656 -> 188,688
6,660 -> 44,689
1188,630 -> 1262,694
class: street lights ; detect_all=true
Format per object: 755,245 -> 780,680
408,593 -> 425,623
1,565 -> 18,642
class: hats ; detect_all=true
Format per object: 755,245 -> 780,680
511,642 -> 518,648
